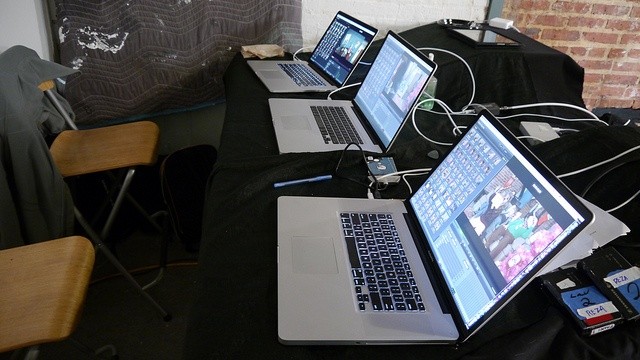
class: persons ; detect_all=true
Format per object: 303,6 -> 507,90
487,214 -> 538,259
496,223 -> 563,283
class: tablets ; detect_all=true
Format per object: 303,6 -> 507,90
448,26 -> 522,48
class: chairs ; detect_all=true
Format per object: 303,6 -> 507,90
0,236 -> 97,359
0,45 -> 171,324
161,144 -> 218,261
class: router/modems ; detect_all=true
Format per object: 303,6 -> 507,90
489,16 -> 515,30
518,120 -> 561,146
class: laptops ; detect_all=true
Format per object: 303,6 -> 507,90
276,107 -> 596,349
266,29 -> 438,156
247,11 -> 378,93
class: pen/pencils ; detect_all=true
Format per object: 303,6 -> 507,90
273,174 -> 333,188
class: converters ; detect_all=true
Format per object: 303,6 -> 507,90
473,102 -> 501,116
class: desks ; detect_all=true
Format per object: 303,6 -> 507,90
198,22 -> 640,358
